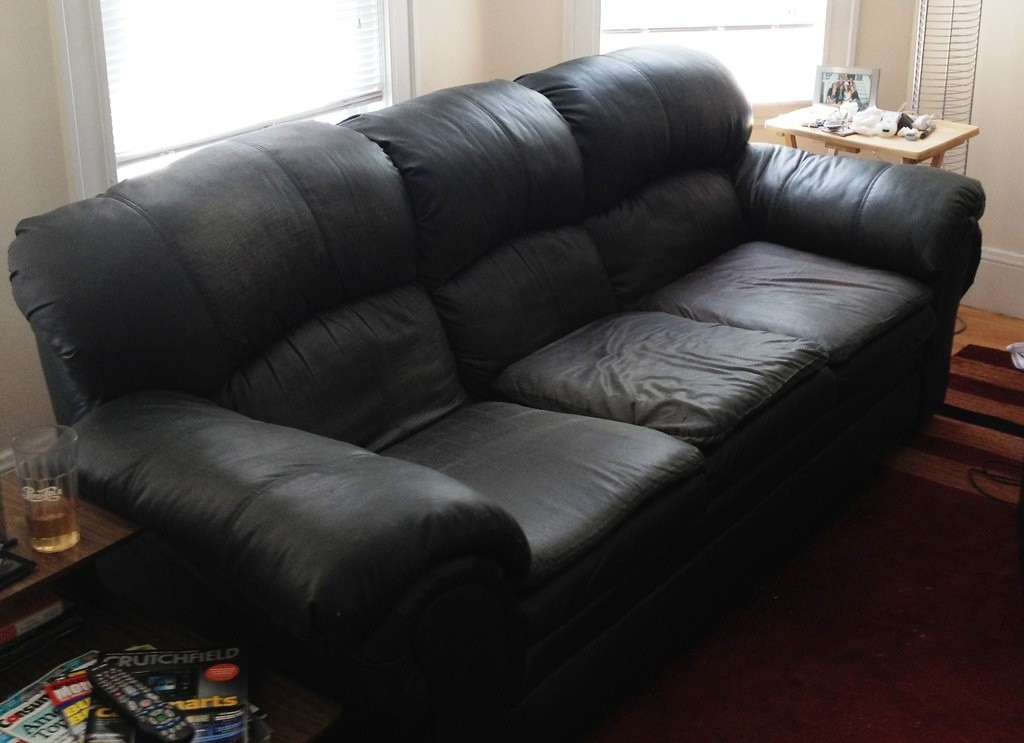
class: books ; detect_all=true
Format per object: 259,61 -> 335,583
0,644 -> 274,743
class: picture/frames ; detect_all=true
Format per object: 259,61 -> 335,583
813,65 -> 879,112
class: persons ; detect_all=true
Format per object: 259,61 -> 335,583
825,76 -> 862,105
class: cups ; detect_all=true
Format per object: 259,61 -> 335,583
12,425 -> 81,552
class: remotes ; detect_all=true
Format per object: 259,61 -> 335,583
85,663 -> 195,743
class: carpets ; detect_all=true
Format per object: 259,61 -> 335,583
301,341 -> 1024,743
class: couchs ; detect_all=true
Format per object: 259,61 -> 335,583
7,39 -> 986,743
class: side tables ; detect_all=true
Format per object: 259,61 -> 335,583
0,470 -> 342,743
763,105 -> 980,171
